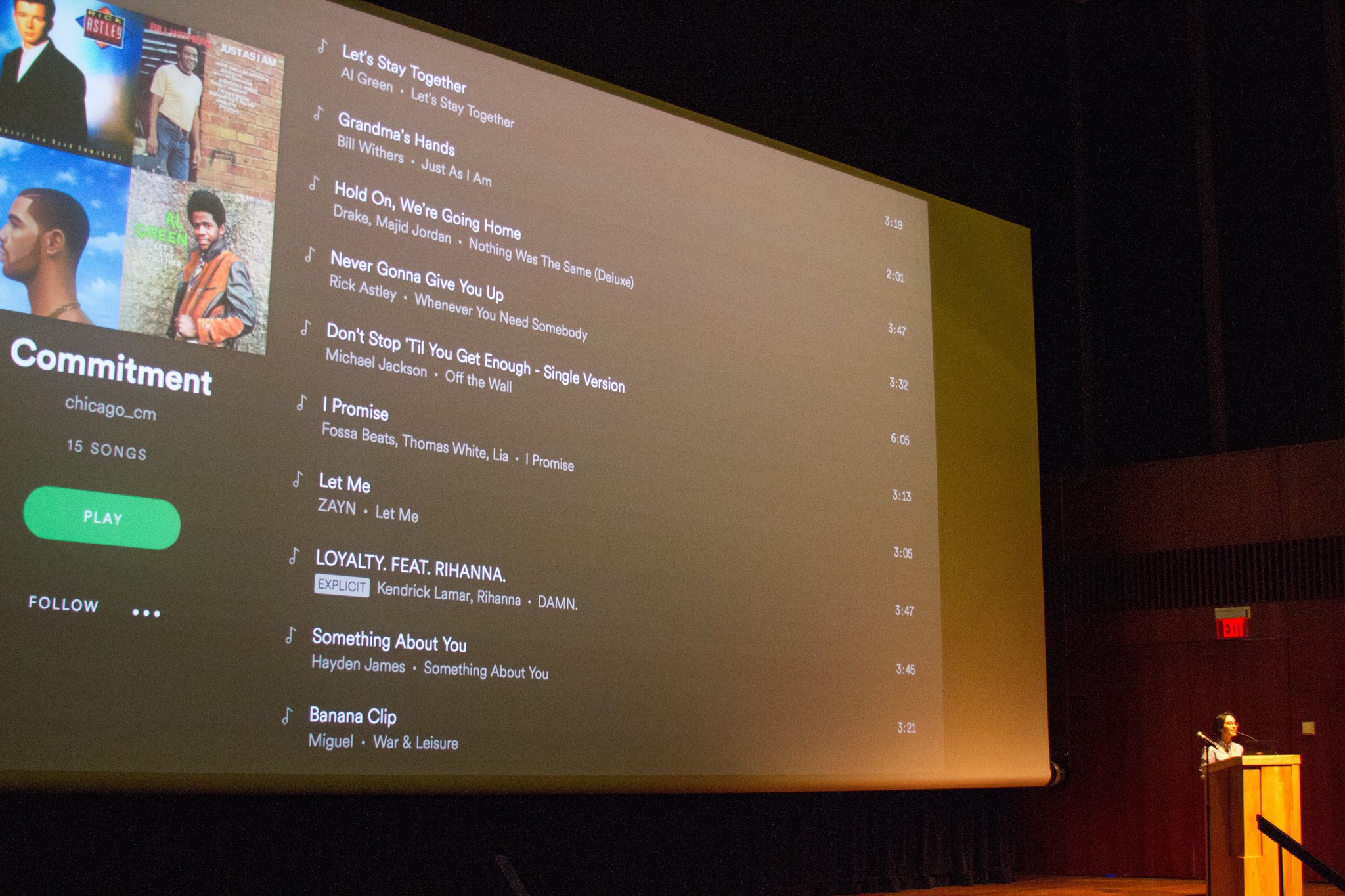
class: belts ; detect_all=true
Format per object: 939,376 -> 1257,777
158,111 -> 189,137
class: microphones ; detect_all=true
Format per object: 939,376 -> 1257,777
1195,731 -> 1219,749
1237,730 -> 1278,754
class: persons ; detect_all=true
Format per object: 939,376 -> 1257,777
165,188 -> 255,348
1198,712 -> 1244,779
0,0 -> 88,154
1,187 -> 96,325
147,40 -> 204,182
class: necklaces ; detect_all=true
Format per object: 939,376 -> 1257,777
48,302 -> 81,320
